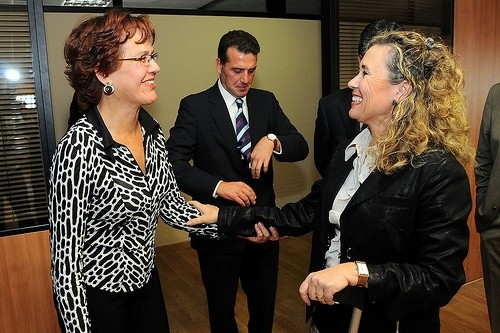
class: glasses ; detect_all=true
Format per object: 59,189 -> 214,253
114,52 -> 159,68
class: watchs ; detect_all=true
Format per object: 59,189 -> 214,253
355,261 -> 369,287
267,133 -> 277,150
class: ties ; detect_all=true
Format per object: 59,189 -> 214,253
235,97 -> 252,165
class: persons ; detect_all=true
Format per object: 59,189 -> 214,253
48,11 -> 288,333
185,31 -> 472,333
166,30 -> 309,333
313,20 -> 441,179
475,83 -> 500,333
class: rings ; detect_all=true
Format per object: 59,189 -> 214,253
316,295 -> 324,300
